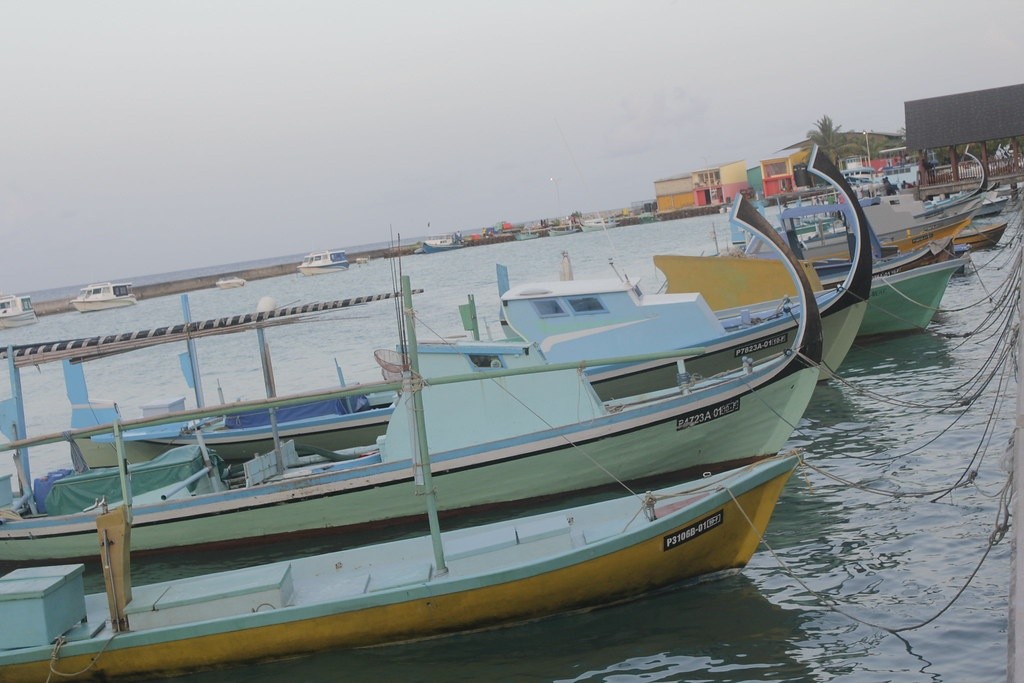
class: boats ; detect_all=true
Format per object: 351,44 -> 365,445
69,280 -> 138,313
1,296 -> 39,334
0,189 -> 824,568
0,446 -> 817,681
341,128 -> 1013,420
216,275 -> 246,290
298,251 -> 350,278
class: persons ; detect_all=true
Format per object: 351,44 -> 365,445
884,180 -> 914,195
921,158 -> 936,185
827,184 -> 863,218
